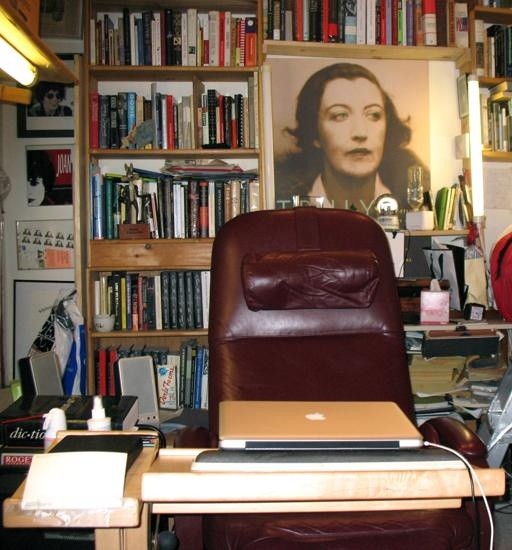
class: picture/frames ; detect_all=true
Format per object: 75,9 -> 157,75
16,49 -> 88,138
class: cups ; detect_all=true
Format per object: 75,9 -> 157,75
93,315 -> 115,333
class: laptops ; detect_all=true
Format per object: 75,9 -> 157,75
219,400 -> 425,451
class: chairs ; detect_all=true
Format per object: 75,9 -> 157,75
170,206 -> 494,549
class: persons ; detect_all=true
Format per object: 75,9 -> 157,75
26,82 -> 70,115
27,151 -> 53,206
284,61 -> 413,214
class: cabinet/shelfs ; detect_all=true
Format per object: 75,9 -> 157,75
74,0 -> 512,412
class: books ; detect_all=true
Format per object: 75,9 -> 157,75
0,392 -> 139,443
471,0 -> 512,153
436,181 -> 462,232
94,337 -> 208,412
89,158 -> 259,241
94,270 -> 210,331
89,0 -> 470,70
408,354 -> 507,419
89,82 -> 259,150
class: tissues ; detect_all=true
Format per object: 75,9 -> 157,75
420,279 -> 450,325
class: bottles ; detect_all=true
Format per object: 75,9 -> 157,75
407,166 -> 423,211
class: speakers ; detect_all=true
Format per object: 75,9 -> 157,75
114,354 -> 160,431
19,351 -> 65,396
